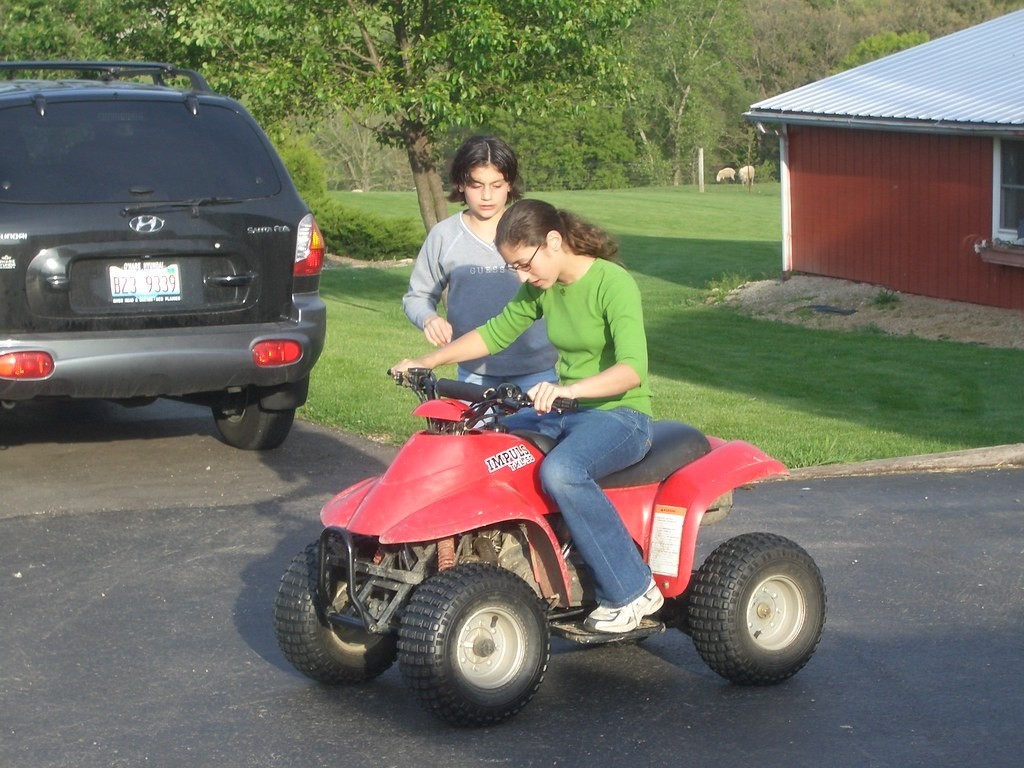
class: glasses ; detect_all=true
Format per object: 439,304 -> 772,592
504,243 -> 541,273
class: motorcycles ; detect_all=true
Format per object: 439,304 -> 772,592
274,368 -> 827,728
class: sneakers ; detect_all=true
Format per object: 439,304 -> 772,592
583,575 -> 664,634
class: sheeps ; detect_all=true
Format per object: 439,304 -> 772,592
716,165 -> 755,186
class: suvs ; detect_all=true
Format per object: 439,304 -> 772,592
0,60 -> 326,451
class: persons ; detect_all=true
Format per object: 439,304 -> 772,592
390,199 -> 663,633
401,135 -> 559,428
